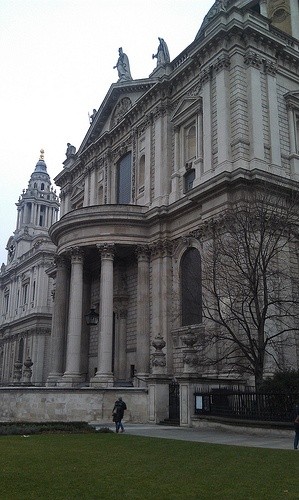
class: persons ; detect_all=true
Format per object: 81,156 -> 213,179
293,413 -> 299,449
112,396 -> 127,432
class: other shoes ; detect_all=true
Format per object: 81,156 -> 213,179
120,430 -> 125,433
115,430 -> 119,433
